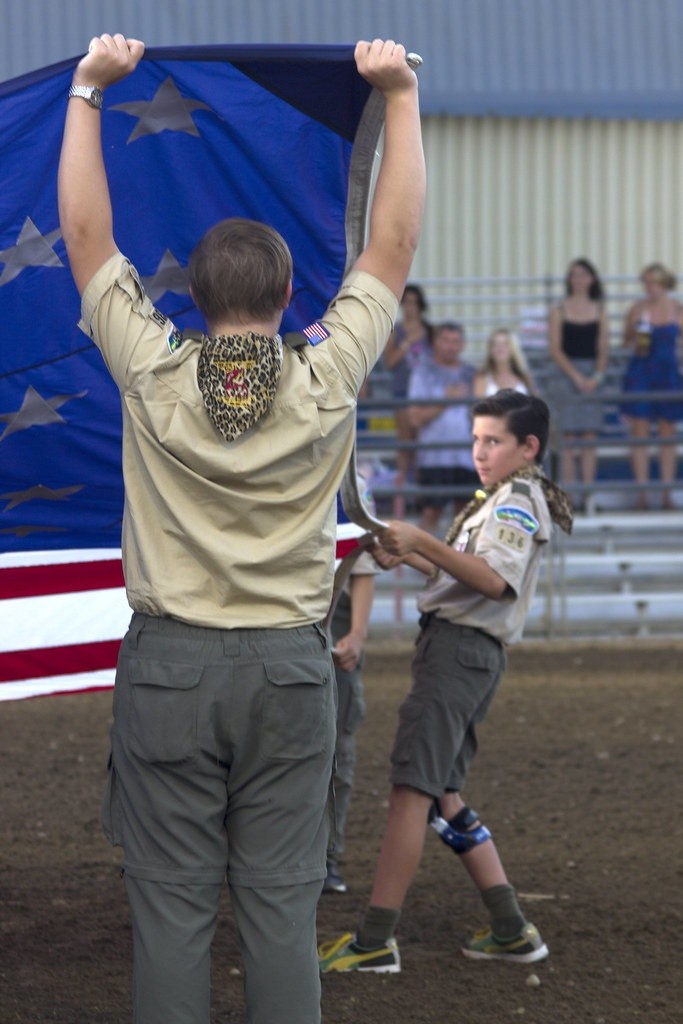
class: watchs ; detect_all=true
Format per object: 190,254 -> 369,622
67,85 -> 103,110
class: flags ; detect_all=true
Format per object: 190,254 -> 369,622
0,43 -> 423,555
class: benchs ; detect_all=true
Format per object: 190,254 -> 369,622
368,272 -> 683,642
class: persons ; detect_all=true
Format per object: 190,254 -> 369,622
327,471 -> 381,892
317,389 -> 574,975
56,32 -> 426,1024
361,257 -> 683,511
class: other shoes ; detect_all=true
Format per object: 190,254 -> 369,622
321,868 -> 346,894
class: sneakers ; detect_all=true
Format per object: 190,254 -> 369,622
318,934 -> 402,973
461,922 -> 548,964
